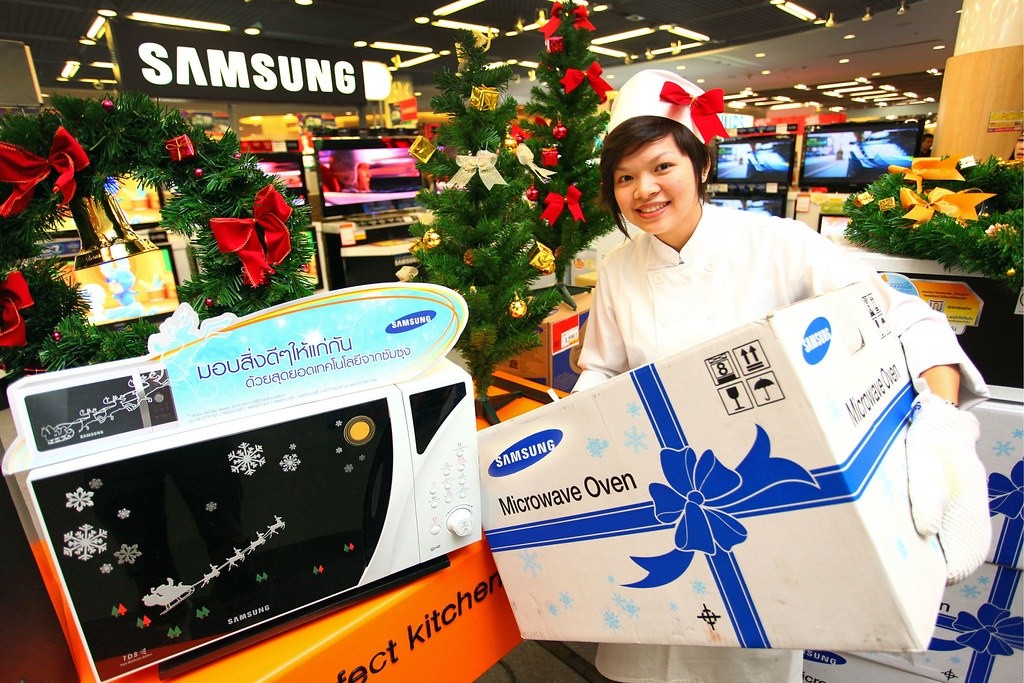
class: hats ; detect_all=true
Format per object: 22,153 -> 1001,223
608,69 -> 731,146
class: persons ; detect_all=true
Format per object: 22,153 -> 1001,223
562,71 -> 959,683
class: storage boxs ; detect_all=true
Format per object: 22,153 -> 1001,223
492,284 -> 594,393
802,385 -> 1023,683
165,371 -> 571,683
476,274 -> 946,653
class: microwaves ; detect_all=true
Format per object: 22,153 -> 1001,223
826,234 -> 1024,404
1,356 -> 483,683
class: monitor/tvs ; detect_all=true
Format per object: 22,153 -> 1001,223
34,170 -> 167,239
312,140 -> 430,217
73,247 -> 182,332
241,152 -> 311,225
704,123 -> 924,237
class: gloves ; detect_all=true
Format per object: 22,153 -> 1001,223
905,378 -> 999,587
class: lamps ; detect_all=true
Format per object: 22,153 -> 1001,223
825,12 -> 836,28
862,6 -> 873,21
896,0 -> 910,16
